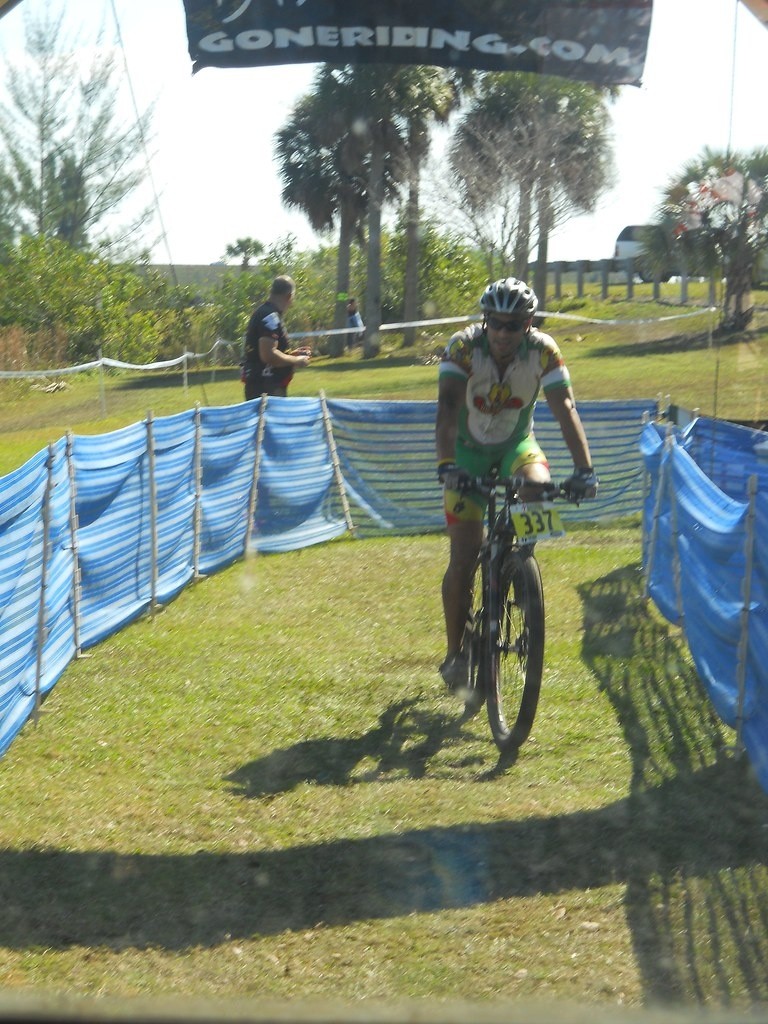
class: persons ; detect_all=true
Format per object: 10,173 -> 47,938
347,298 -> 363,351
435,278 -> 599,683
241,276 -> 311,402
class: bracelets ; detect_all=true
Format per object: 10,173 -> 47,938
438,458 -> 456,465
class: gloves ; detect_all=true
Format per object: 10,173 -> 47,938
566,466 -> 598,499
446,466 -> 476,495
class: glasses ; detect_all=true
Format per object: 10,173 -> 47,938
487,317 -> 527,332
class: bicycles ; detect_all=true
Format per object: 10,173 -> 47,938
448,466 -> 589,753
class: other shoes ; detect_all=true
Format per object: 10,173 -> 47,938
444,652 -> 469,694
513,571 -> 529,609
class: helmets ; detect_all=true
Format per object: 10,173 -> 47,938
479,277 -> 539,316
